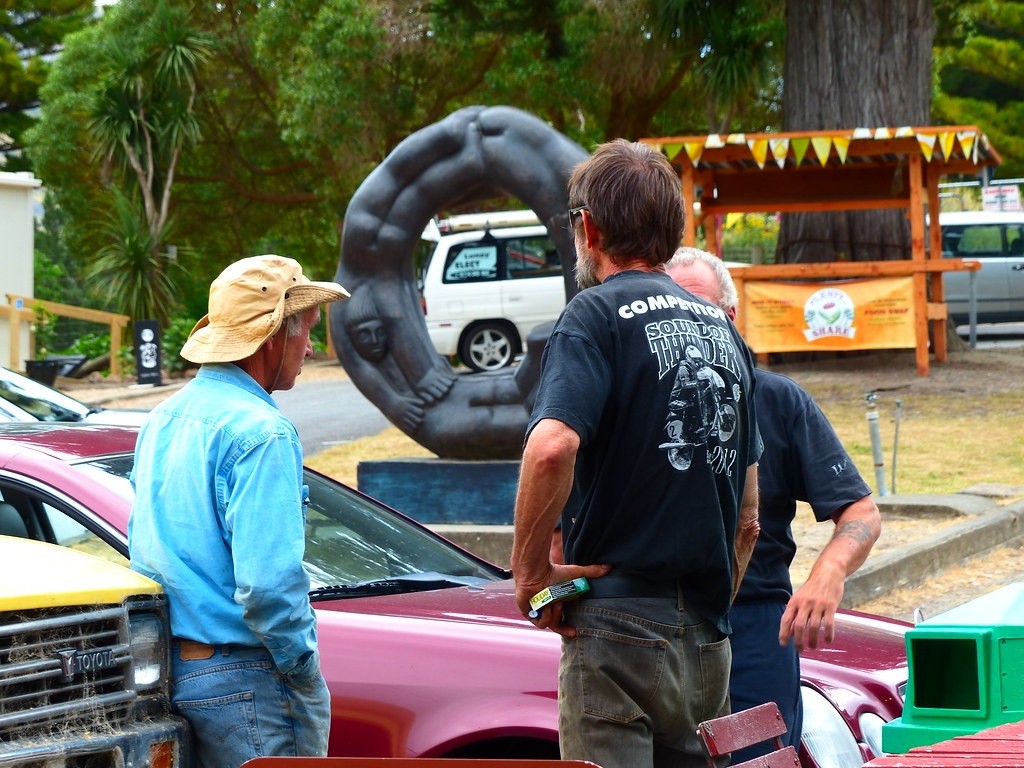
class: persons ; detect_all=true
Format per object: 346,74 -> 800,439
661,248 -> 882,768
128,254 -> 351,768
326,106 -> 602,460
510,138 -> 765,768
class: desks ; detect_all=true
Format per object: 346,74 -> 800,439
862,720 -> 1023,768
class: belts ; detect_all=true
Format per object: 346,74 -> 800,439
579,575 -> 686,599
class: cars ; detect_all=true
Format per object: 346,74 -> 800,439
0,367 -> 926,768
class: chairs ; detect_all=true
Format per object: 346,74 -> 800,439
697,702 -> 802,768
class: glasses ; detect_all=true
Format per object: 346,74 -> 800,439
568,205 -> 594,228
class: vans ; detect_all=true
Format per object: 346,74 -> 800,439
420,222 -> 566,371
923,210 -> 1024,335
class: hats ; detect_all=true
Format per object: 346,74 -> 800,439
180,254 -> 351,364
